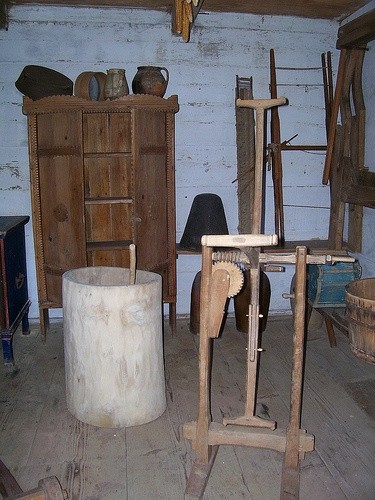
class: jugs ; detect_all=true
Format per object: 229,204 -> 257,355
104,68 -> 129,101
132,66 -> 170,97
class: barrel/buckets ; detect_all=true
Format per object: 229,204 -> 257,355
344,278 -> 375,366
63,266 -> 166,428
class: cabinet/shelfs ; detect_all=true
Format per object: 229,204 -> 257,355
21,93 -> 179,345
0,214 -> 31,366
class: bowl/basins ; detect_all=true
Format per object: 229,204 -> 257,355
15,66 -> 73,101
